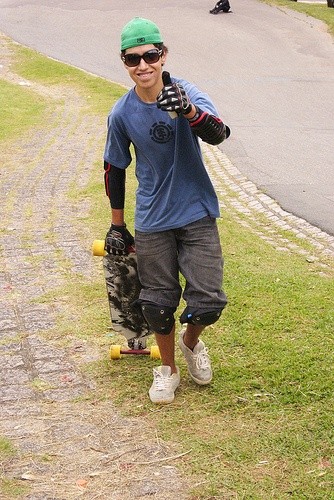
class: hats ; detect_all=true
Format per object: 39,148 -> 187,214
120,16 -> 164,50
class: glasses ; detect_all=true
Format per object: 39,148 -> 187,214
123,48 -> 163,68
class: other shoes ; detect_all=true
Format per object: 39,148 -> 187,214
208,10 -> 217,14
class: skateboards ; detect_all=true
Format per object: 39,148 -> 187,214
92,224 -> 161,359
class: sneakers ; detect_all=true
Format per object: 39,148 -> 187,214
179,330 -> 213,385
148,365 -> 180,404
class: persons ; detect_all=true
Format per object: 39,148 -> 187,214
103,18 -> 232,405
209,0 -> 230,14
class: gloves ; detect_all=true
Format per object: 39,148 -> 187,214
104,223 -> 134,259
156,70 -> 192,115
